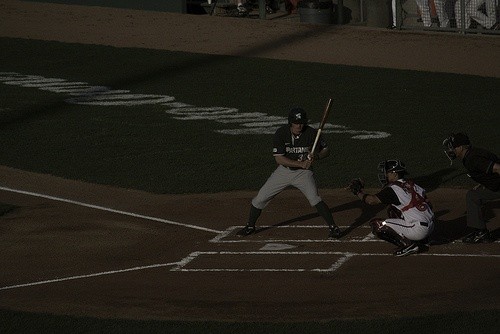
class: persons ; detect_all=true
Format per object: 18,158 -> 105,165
237,108 -> 341,239
443,133 -> 500,243
416,0 -> 497,30
344,160 -> 435,256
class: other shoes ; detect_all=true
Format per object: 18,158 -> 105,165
237,5 -> 246,12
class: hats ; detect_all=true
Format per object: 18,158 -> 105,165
451,136 -> 470,149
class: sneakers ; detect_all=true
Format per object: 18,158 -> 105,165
394,242 -> 418,257
235,224 -> 255,236
461,230 -> 490,243
329,224 -> 340,238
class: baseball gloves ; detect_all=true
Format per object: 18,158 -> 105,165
345,178 -> 365,198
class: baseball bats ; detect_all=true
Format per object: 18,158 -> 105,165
307,98 -> 334,161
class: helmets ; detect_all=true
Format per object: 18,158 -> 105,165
288,109 -> 307,126
386,159 -> 408,173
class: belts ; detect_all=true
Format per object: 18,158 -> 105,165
283,165 -> 301,170
420,222 -> 428,227
